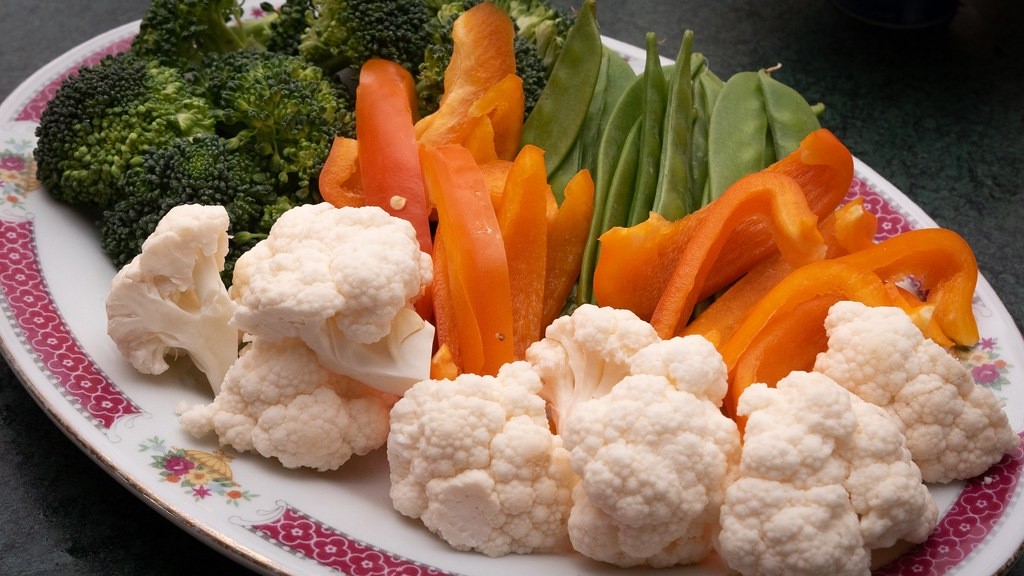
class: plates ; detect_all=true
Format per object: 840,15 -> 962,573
0,0 -> 1023,576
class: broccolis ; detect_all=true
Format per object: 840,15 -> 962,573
103,203 -> 1022,575
32,1 -> 579,287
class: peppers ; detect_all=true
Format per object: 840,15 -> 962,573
414,1 -> 517,147
320,61 -> 980,436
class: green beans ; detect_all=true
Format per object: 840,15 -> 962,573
518,1 -> 825,313
